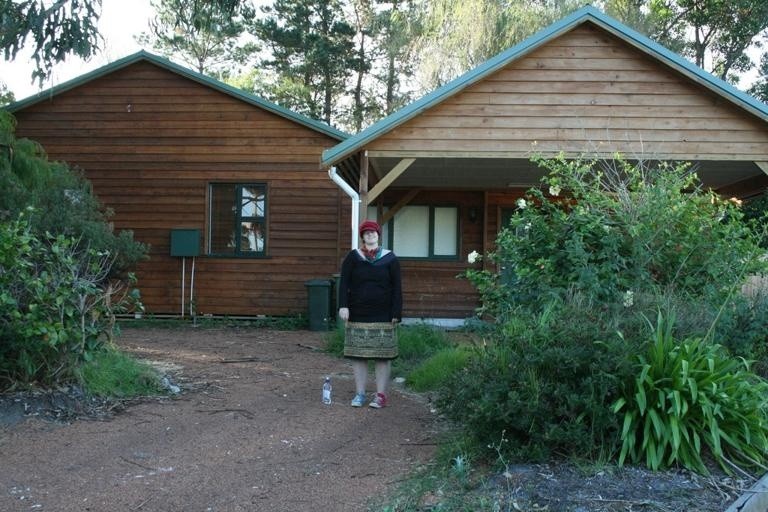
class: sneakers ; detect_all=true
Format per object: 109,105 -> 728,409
351,393 -> 366,407
369,392 -> 387,409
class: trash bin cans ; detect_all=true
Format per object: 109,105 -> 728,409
304,280 -> 335,331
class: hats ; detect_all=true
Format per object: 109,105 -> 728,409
360,221 -> 380,238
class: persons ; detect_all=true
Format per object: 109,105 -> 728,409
336,221 -> 404,408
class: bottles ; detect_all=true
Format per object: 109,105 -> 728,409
321,376 -> 334,405
